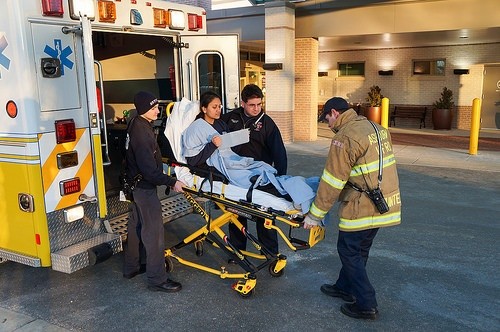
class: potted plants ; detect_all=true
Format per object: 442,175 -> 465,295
432,87 -> 453,129
365,85 -> 381,125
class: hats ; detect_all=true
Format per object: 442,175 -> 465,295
134,91 -> 158,115
318,97 -> 349,122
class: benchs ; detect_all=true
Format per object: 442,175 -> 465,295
390,106 -> 427,129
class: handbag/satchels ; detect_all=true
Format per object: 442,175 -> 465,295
119,169 -> 141,200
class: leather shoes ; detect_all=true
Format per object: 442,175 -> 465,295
123,263 -> 146,279
148,278 -> 182,292
228,258 -> 237,264
341,303 -> 379,319
321,284 -> 356,302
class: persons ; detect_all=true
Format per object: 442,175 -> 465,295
301,97 -> 401,320
122,92 -> 182,292
178,90 -> 297,202
218,83 -> 288,259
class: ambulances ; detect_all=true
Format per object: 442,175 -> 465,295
0,0 -> 241,275
